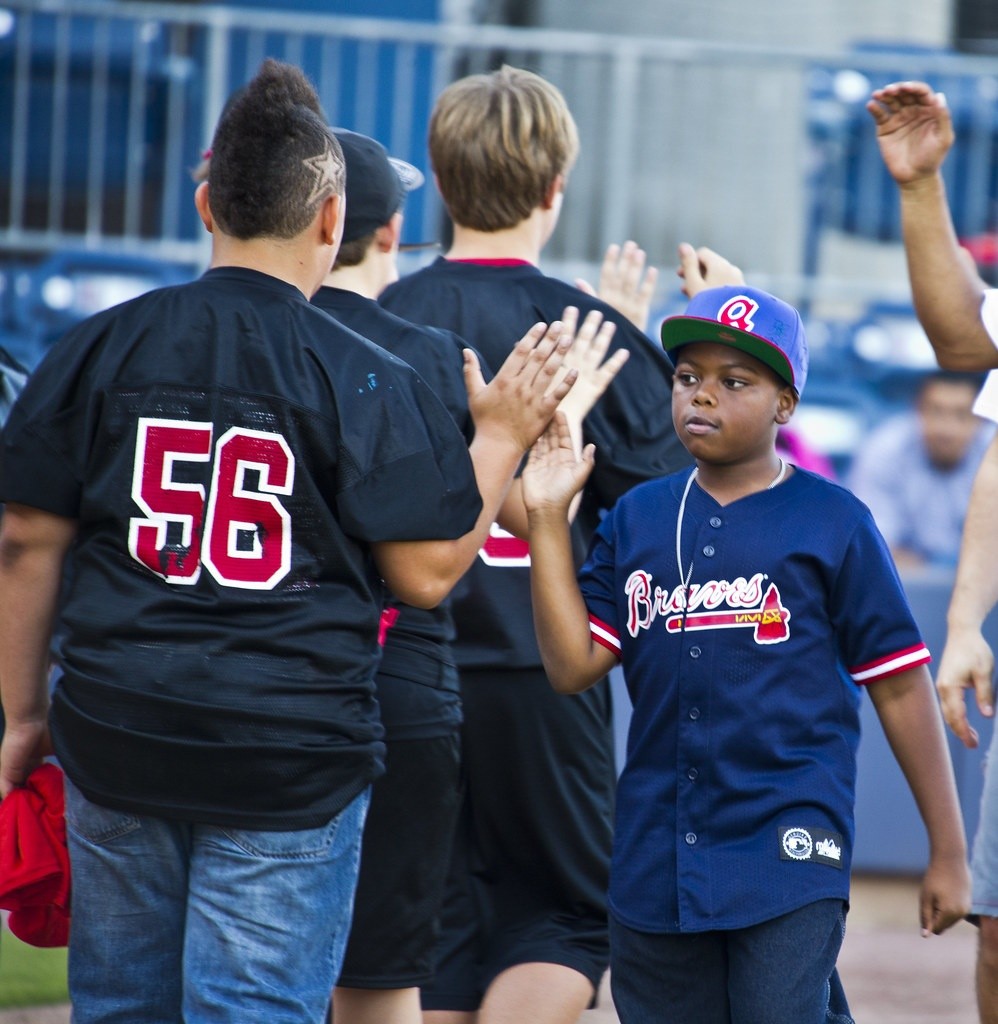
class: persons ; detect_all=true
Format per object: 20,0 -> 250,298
520,285 -> 974,1024
1,58 -> 578,1023
308,122 -> 632,1023
848,80 -> 998,1024
379,66 -> 745,1024
574,242 -> 659,331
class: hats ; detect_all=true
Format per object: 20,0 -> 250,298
327,125 -> 427,240
660,287 -> 808,400
